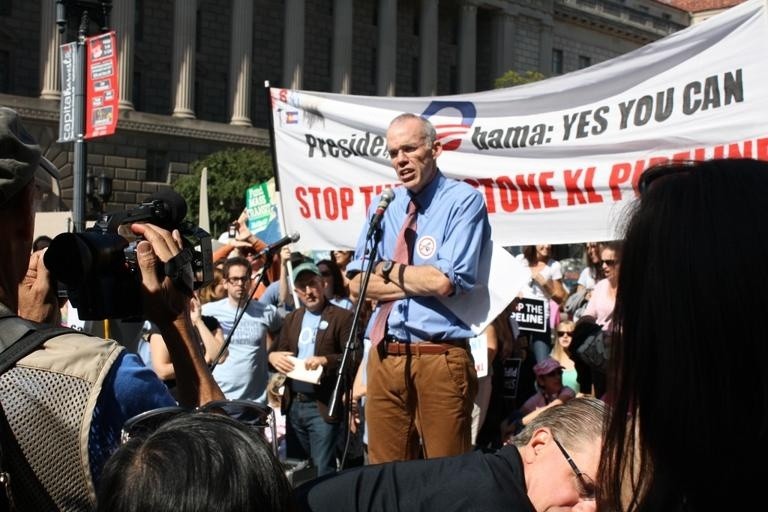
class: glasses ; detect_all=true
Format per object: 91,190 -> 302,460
557,331 -> 572,336
599,260 -> 615,265
554,438 -> 593,497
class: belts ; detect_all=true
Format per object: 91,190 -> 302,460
293,392 -> 312,402
383,339 -> 466,354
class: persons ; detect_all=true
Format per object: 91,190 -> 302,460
95,415 -> 296,511
21,208 -> 372,467
293,397 -> 644,512
466,157 -> 766,511
0,108 -> 227,511
345,113 -> 491,465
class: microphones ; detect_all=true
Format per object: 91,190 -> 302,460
366,188 -> 398,241
252,230 -> 302,262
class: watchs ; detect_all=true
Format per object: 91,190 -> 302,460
382,260 -> 396,282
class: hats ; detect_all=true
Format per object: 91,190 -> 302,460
533,358 -> 565,376
293,262 -> 320,283
0,107 -> 61,204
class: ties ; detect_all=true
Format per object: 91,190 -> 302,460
370,201 -> 417,347
